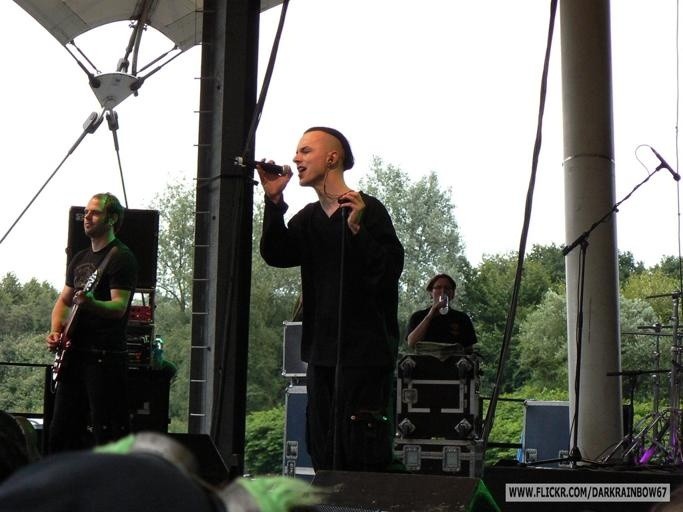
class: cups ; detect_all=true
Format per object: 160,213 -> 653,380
439,295 -> 448,314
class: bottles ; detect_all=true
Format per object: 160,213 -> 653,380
152,334 -> 163,359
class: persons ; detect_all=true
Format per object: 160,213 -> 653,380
405,273 -> 477,347
46,193 -> 139,453
257,126 -> 404,472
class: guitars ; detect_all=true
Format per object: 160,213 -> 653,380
50,269 -> 101,380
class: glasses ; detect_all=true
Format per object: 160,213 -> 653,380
431,285 -> 453,290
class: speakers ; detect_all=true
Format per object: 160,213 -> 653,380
66,207 -> 160,291
311,469 -> 501,512
43,364 -> 171,455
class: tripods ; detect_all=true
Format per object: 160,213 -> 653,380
517,162 -> 683,471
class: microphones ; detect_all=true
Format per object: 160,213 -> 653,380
233,156 -> 292,177
651,147 -> 681,182
